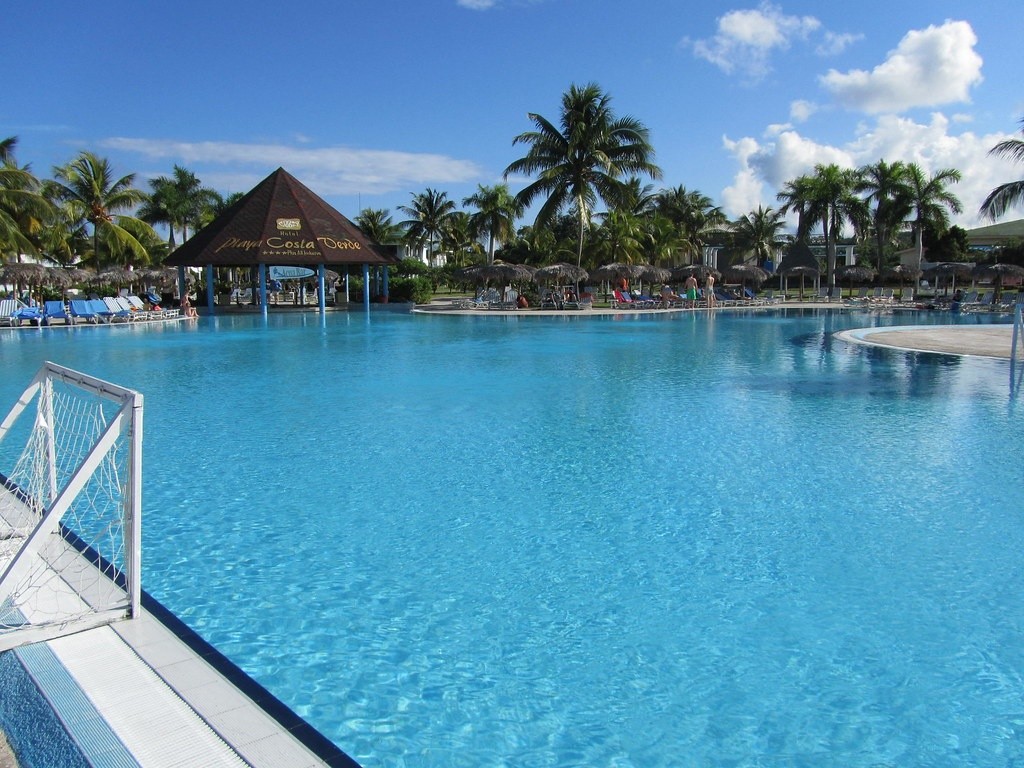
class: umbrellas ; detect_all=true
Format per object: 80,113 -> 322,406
591,262 -> 672,296
723,262 -> 772,298
881,264 -> 924,300
776,245 -> 824,302
972,263 -> 1024,301
672,263 -> 721,280
0,263 -> 196,308
312,270 -> 338,282
455,259 -> 589,295
834,265 -> 874,296
923,263 -> 974,293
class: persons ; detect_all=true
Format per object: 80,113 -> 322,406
144,290 -> 162,310
953,290 -> 962,302
516,293 -> 529,308
566,290 -> 576,302
682,273 -> 698,309
621,277 -> 627,292
269,279 -> 282,304
180,291 -> 199,317
702,273 -> 715,307
550,287 -> 560,306
658,285 -> 686,301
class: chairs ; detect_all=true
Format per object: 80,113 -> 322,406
126,295 -> 163,320
933,290 -> 1024,313
0,299 -> 21,326
147,292 -> 181,317
541,289 -> 593,310
139,293 -> 176,319
116,296 -> 149,322
88,299 -> 131,324
231,288 -> 260,302
69,299 -> 99,325
103,297 -> 136,323
809,287 -> 914,308
451,289 -> 519,311
920,280 -> 933,296
44,301 -> 73,326
15,306 -> 42,328
607,289 -> 785,309
